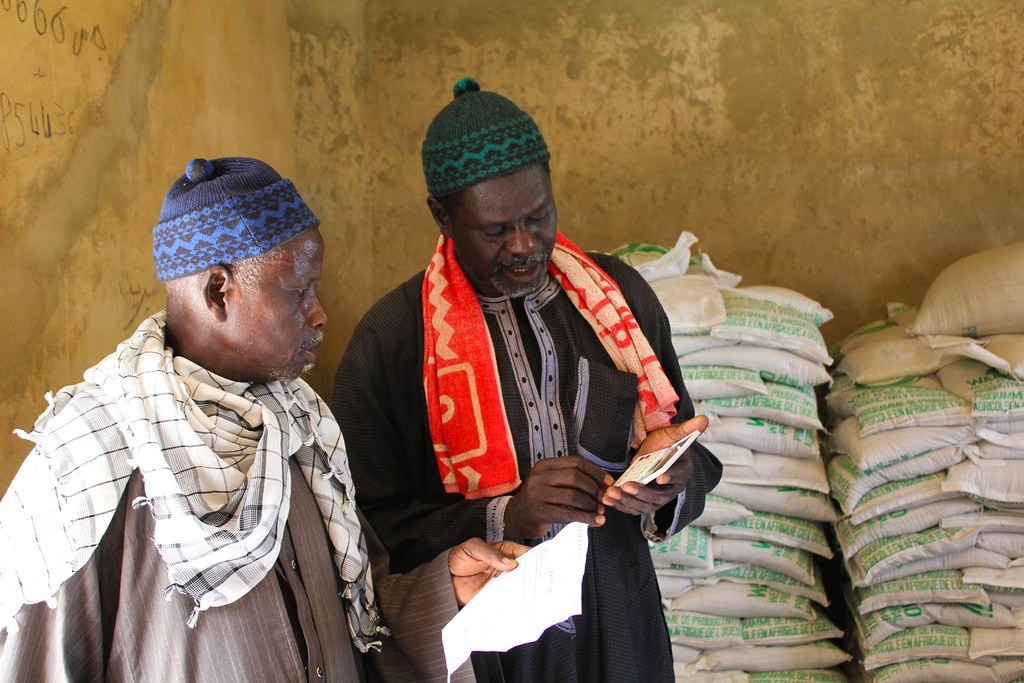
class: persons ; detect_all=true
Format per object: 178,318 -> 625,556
331,75 -> 723,681
0,158 -> 535,682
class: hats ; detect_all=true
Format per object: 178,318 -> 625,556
152,156 -> 320,282
422,78 -> 551,198
